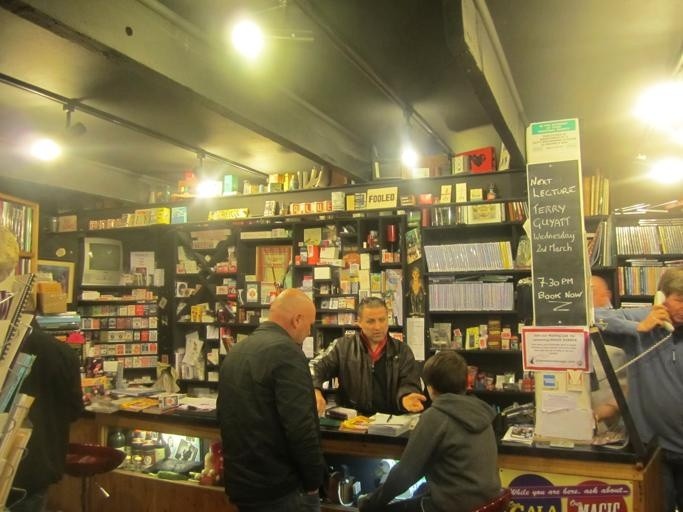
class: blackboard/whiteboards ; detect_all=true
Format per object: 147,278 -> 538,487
527,160 -> 588,327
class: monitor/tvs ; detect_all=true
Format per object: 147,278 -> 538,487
81,237 -> 123,286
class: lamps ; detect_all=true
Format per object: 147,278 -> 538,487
48,108 -> 88,148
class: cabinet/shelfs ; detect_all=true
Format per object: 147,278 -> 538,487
583,170 -> 620,310
0,167 -> 534,428
615,212 -> 683,310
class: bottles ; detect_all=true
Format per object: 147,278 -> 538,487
158,369 -> 174,411
105,428 -> 170,477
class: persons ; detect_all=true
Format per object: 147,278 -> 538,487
213,286 -> 330,510
590,267 -> 683,512
304,296 -> 427,415
353,349 -> 501,511
576,271 -> 633,432
5,315 -> 85,511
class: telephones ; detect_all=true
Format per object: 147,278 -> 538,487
654,291 -> 675,333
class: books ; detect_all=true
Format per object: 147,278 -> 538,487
583,175 -> 609,217
420,201 -> 528,311
614,224 -> 683,295
500,425 -> 535,449
0,201 -> 40,511
80,385 -> 216,417
509,423 -> 534,440
